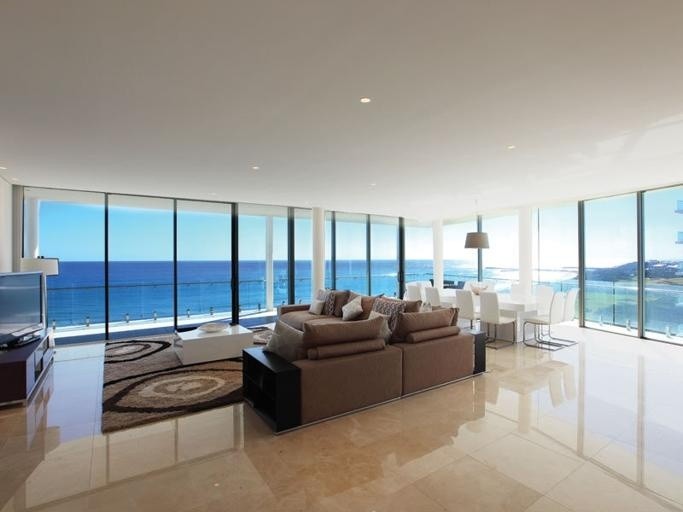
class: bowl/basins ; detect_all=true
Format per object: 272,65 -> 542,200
471,283 -> 488,296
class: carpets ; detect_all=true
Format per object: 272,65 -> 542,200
98,325 -> 274,435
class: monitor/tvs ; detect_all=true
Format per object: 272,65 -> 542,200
0,272 -> 48,349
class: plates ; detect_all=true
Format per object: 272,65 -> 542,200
198,323 -> 229,333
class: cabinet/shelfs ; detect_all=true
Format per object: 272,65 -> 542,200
0,325 -> 56,408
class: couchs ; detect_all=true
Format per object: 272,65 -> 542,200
238,304 -> 487,436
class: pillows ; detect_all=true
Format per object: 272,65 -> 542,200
260,316 -> 307,364
306,285 -> 434,344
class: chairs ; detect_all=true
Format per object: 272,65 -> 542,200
404,276 -> 582,354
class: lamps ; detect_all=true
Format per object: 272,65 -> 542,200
461,199 -> 489,250
15,254 -> 60,349
